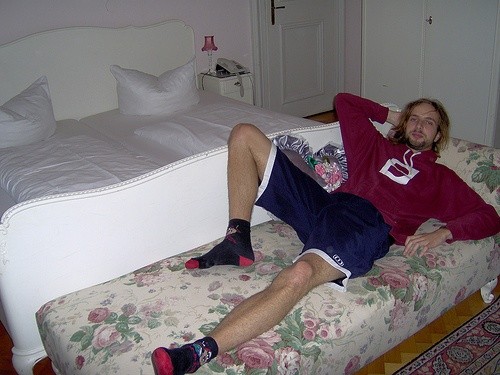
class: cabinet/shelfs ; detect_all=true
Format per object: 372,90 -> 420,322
361,0 -> 500,149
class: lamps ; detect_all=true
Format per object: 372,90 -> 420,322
202,36 -> 217,73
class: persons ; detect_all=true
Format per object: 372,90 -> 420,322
151,93 -> 500,375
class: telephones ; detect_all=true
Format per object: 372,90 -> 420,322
216,58 -> 245,75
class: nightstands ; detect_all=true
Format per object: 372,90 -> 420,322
198,69 -> 253,106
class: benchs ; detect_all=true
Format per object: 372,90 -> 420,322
35,136 -> 500,375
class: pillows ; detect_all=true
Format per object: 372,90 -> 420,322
0,74 -> 56,149
108,56 -> 199,117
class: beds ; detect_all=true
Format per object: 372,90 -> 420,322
0,20 -> 400,375
